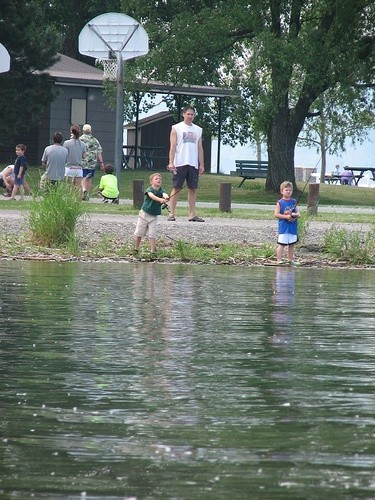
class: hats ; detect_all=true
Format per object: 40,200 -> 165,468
82,124 -> 91,132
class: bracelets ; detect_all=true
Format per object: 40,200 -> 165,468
100,162 -> 103,163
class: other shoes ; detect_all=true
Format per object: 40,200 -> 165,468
277,261 -> 285,264
189,216 -> 205,222
130,250 -> 139,255
151,252 -> 157,255
8,197 -> 16,200
111,197 -> 119,204
287,261 -> 294,265
82,189 -> 89,200
102,199 -> 109,204
2,193 -> 11,197
167,217 -> 175,221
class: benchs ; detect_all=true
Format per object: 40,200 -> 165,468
325,175 -> 364,187
235,160 -> 267,188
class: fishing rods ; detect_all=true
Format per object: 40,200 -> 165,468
160,165 -> 202,213
288,133 -> 342,223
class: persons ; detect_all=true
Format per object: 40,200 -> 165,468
276,181 -> 300,264
40,124 -> 104,201
0,165 -> 33,198
169,106 -> 205,221
334,165 -> 352,184
98,165 -> 120,203
8,144 -> 27,201
134,173 -> 169,259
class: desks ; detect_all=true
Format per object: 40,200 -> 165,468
344,167 -> 375,187
